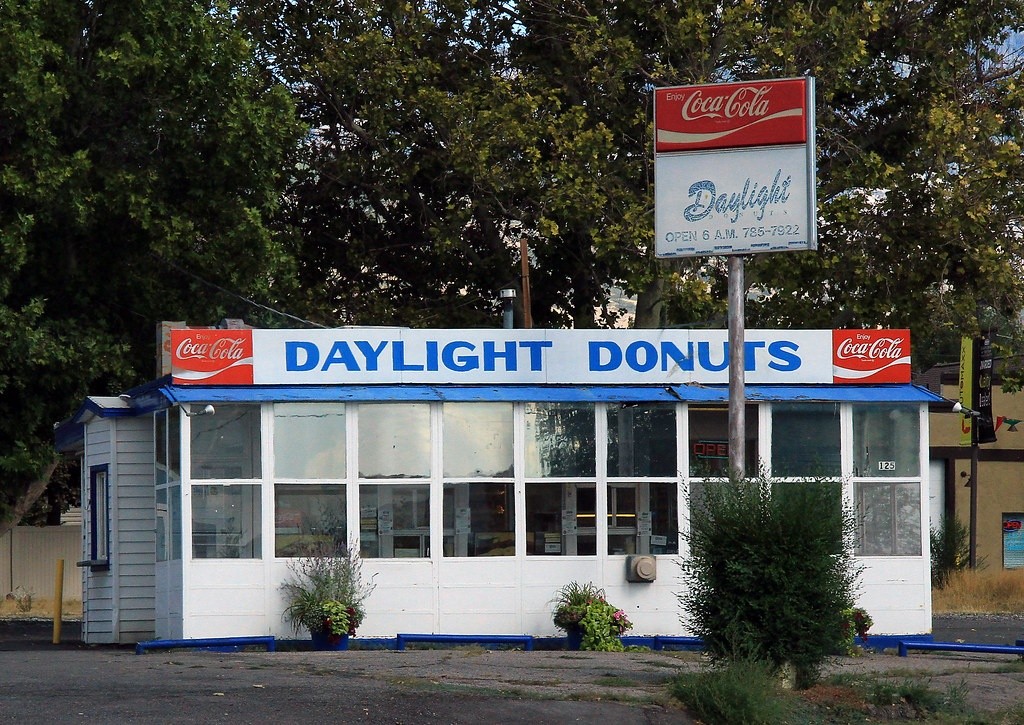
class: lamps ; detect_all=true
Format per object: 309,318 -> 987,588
910,383 -> 982,418
161,385 -> 216,417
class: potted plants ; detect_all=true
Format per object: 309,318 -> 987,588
280,538 -> 379,650
564,625 -> 588,650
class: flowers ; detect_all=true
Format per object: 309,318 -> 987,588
550,581 -> 634,651
